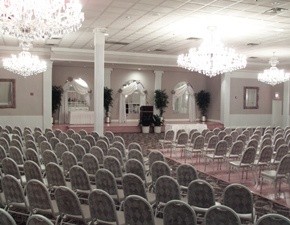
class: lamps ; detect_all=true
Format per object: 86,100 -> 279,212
177,39 -> 247,78
0,0 -> 85,78
258,54 -> 290,86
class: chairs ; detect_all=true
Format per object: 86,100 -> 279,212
0,125 -> 290,225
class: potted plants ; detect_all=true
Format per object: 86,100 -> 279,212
195,90 -> 210,122
138,88 -> 171,134
104,86 -> 113,123
